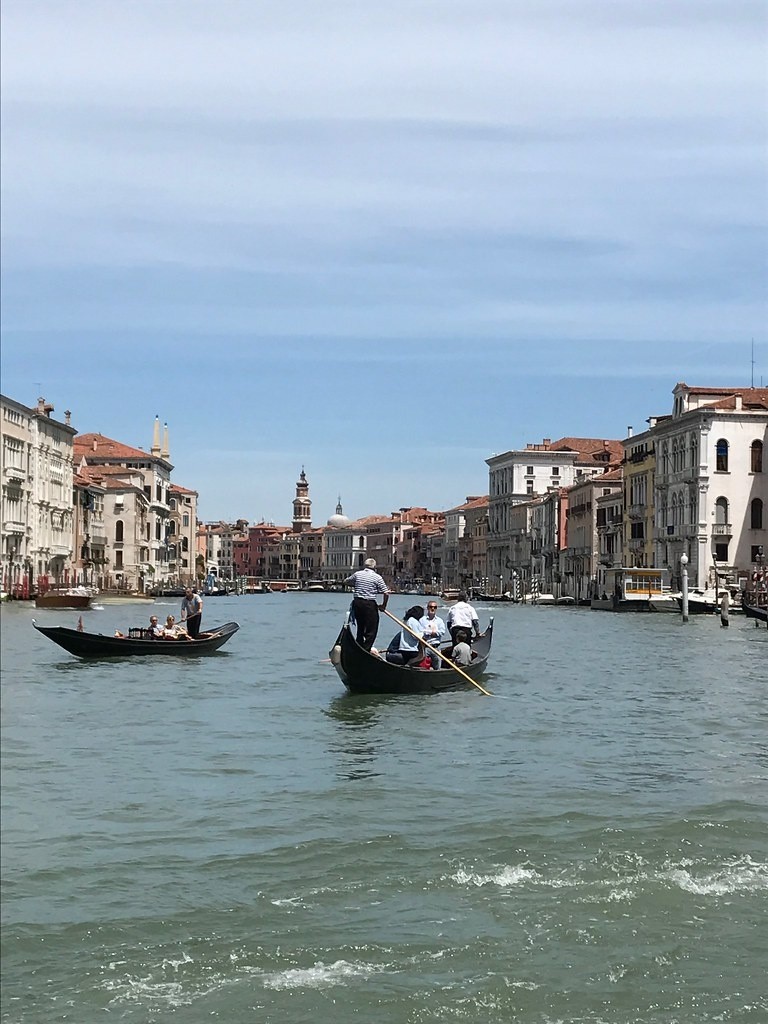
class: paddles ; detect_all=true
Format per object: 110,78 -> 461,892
173,610 -> 200,626
382,608 -> 490,695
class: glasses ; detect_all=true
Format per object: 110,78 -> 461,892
428,605 -> 437,610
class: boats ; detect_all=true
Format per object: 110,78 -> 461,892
328,612 -> 494,694
149,571 -> 582,607
36,586 -> 95,610
589,566 -> 668,612
671,584 -> 744,615
31,619 -> 241,661
649,587 -> 701,612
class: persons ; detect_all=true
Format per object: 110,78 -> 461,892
398,600 -> 446,670
164,614 -> 192,640
447,591 -> 480,649
451,629 -> 472,667
181,589 -> 204,635
148,615 -> 165,638
343,557 -> 389,652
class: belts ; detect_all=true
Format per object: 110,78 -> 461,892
432,643 -> 440,648
353,597 -> 367,601
